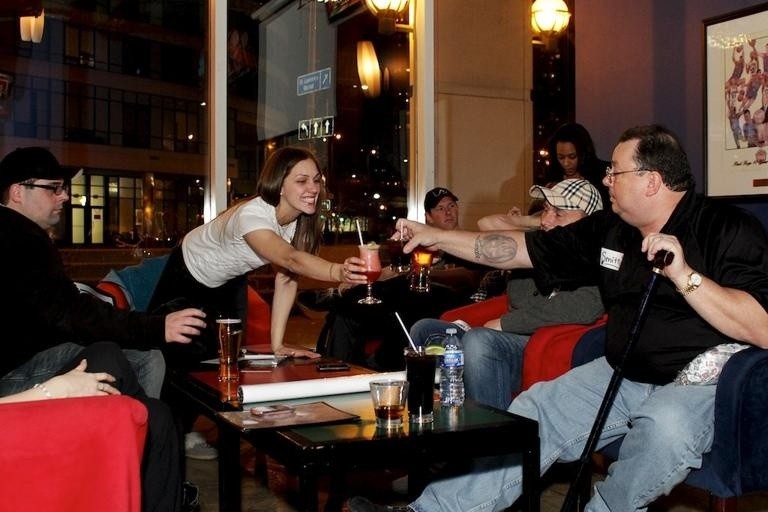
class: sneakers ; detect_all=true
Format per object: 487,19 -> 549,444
346,495 -> 412,512
185,432 -> 217,461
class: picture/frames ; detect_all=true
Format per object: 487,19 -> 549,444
701,3 -> 768,199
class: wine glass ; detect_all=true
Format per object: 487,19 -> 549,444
357,243 -> 384,304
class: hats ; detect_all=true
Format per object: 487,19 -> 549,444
528,178 -> 604,216
1,146 -> 84,186
424,187 -> 459,209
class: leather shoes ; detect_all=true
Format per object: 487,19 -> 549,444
298,291 -> 336,311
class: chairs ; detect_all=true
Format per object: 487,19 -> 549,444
0,394 -> 149,512
570,322 -> 767,512
97,254 -> 272,445
439,292 -> 608,403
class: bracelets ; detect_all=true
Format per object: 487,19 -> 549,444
330,263 -> 335,281
33,383 -> 53,398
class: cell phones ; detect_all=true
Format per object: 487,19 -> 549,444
250,403 -> 294,415
316,362 -> 349,371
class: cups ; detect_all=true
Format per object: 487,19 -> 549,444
216,315 -> 242,382
385,238 -> 411,275
409,247 -> 432,290
403,344 -> 440,427
369,379 -> 410,429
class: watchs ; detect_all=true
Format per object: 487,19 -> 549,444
676,270 -> 702,296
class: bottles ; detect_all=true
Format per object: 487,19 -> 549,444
439,328 -> 465,407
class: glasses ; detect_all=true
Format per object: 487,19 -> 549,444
606,166 -> 653,184
19,183 -> 71,196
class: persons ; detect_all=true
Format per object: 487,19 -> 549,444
408,177 -> 607,412
146,147 -> 368,358
0,146 -> 207,401
340,123 -> 768,512
0,339 -> 182,511
527,123 -> 611,216
424,187 -> 458,230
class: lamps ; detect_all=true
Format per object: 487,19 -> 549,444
531,0 -> 572,50
356,40 -> 390,98
20,9 -> 46,44
366,0 -> 409,35
